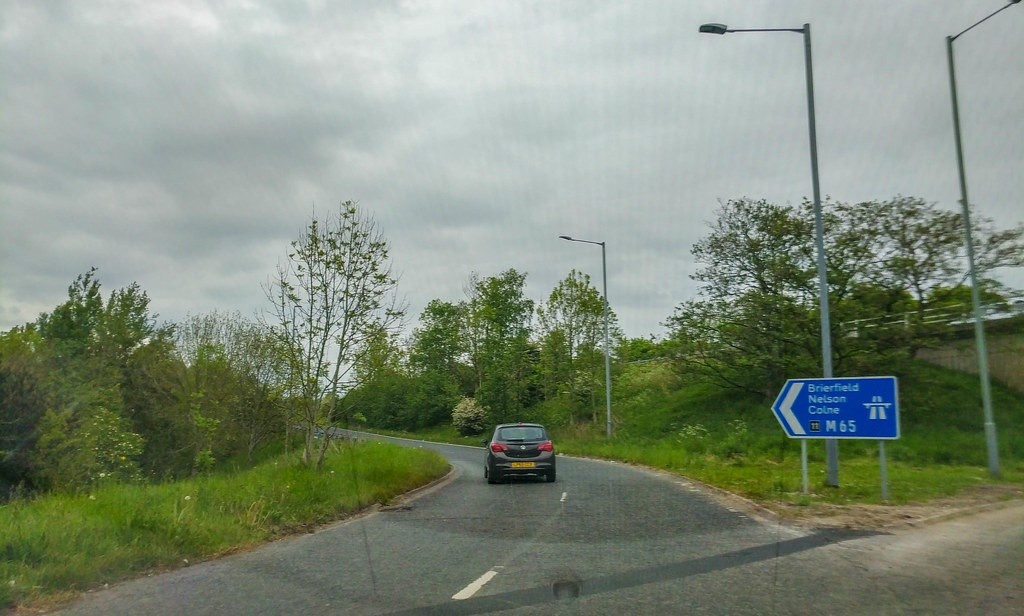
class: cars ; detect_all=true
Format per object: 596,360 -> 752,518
484,422 -> 556,483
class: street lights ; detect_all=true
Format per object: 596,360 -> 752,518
698,20 -> 843,489
559,235 -> 613,438
946,1 -> 1023,477
